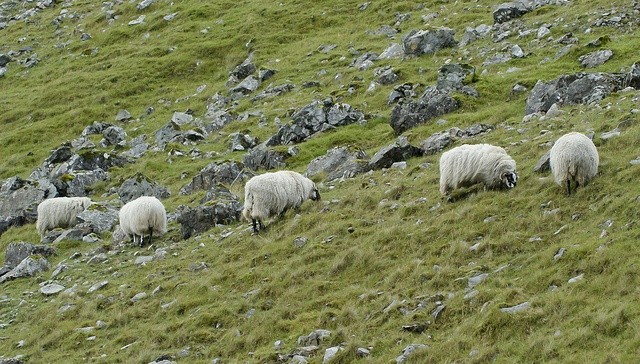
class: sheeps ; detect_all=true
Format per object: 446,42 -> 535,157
119,196 -> 167,247
549,132 -> 599,196
36,197 -> 91,242
439,143 -> 520,196
243,171 -> 321,233
